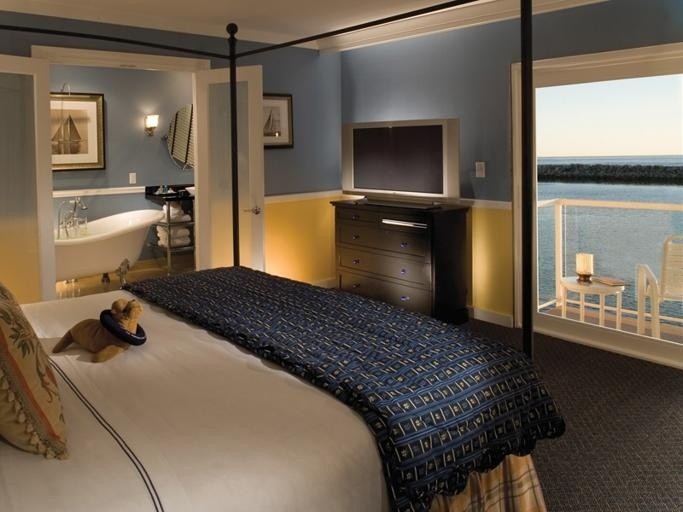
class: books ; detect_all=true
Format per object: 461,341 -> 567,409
592,277 -> 632,286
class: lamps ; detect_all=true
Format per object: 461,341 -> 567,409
574,251 -> 595,287
144,113 -> 160,137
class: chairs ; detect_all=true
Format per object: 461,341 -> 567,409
632,229 -> 683,340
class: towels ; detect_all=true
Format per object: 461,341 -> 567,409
148,198 -> 197,251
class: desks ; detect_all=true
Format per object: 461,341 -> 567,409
555,275 -> 627,331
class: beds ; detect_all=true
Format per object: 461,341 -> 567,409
0,262 -> 569,511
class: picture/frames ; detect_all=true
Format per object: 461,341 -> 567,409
48,89 -> 108,174
261,90 -> 296,152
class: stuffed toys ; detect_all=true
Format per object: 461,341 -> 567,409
52,299 -> 147,362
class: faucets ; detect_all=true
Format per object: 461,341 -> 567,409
72,197 -> 88,218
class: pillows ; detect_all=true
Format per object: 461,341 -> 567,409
0,281 -> 70,464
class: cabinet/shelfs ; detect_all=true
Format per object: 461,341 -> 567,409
328,195 -> 472,327
147,199 -> 195,278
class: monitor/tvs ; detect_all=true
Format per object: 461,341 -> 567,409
341,119 -> 459,210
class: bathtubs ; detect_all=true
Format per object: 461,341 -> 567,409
54,209 -> 165,286
